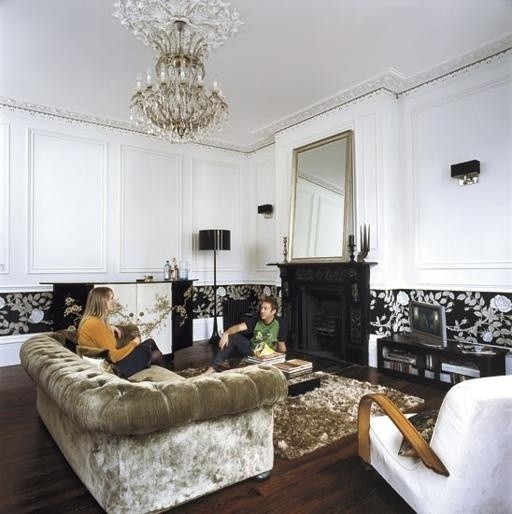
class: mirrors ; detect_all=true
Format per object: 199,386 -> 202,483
288,130 -> 354,263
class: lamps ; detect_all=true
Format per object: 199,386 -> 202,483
258,204 -> 273,219
111,0 -> 244,144
451,159 -> 480,186
199,229 -> 231,348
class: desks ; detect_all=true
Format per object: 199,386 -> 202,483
377,333 -> 512,391
39,278 -> 199,355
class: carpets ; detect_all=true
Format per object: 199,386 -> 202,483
174,357 -> 425,461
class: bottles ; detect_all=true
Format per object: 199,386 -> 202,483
165,257 -> 188,281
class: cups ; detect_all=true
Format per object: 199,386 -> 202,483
144,273 -> 153,283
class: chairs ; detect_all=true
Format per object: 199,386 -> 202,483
357,375 -> 512,514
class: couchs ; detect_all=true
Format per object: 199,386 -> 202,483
20,324 -> 288,514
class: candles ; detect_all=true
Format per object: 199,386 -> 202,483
349,235 -> 354,246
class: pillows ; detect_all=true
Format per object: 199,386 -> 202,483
398,407 -> 439,459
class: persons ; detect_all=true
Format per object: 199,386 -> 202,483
75,286 -> 165,378
204,297 -> 288,373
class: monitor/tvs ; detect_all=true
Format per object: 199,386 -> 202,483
408,300 -> 447,349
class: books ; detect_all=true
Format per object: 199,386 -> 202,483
248,352 -> 313,375
